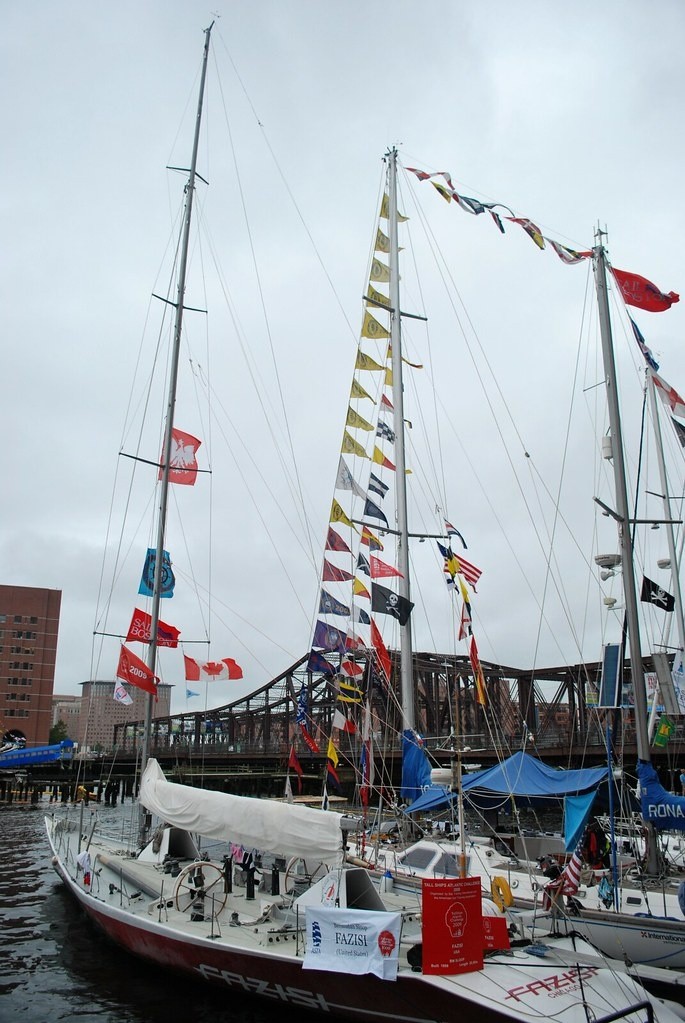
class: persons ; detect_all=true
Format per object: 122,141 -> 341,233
680,769 -> 685,796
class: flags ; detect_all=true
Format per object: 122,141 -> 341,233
286,620 -> 363,811
653,715 -> 675,748
542,823 -> 589,911
138,548 -> 175,598
126,608 -> 180,649
113,677 -> 132,706
158,425 -> 201,486
370,619 -> 391,679
301,905 -> 401,981
117,642 -> 161,703
641,574 -> 675,612
607,264 -> 685,448
437,517 -> 487,707
371,582 -> 415,626
183,651 -> 243,681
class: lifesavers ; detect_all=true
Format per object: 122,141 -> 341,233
491,875 -> 514,913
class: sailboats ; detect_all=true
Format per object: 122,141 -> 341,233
37,17 -> 685,1022
